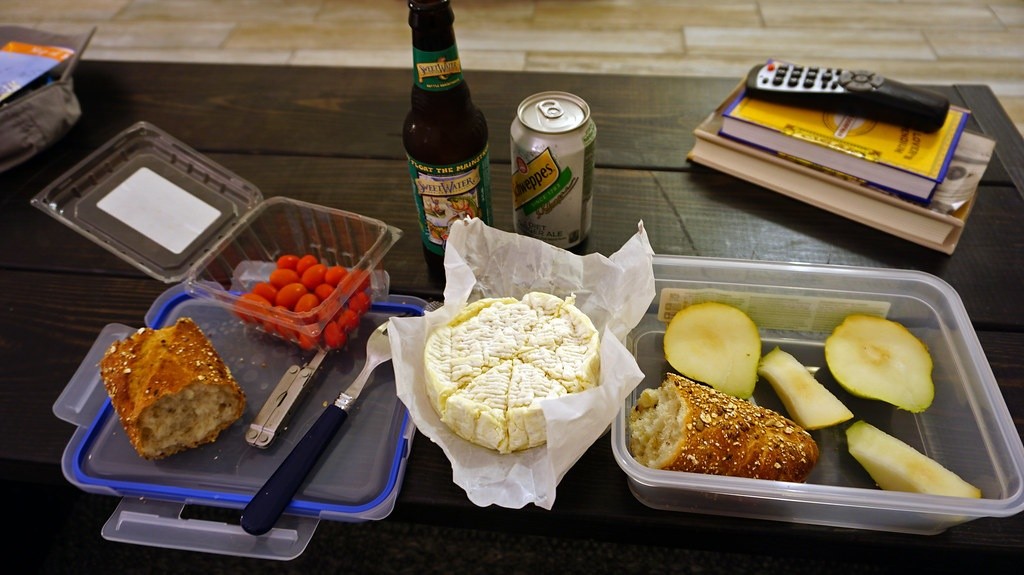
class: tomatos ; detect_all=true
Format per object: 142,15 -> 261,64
233,254 -> 372,352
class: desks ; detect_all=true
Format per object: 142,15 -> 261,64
2,55 -> 1023,575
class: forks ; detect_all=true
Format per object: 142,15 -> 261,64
239,312 -> 421,536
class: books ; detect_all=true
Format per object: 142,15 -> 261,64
718,91 -> 971,203
685,70 -> 997,256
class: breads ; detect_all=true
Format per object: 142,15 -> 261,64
628,372 -> 819,512
97,317 -> 246,464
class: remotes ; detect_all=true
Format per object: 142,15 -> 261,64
744,64 -> 951,134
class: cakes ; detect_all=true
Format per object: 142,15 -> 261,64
422,291 -> 600,455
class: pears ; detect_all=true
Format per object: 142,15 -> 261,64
663,300 -> 983,521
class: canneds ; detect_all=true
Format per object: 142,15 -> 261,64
509,91 -> 598,250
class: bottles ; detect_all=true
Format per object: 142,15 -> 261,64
400,1 -> 494,292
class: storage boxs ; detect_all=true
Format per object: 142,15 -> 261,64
607,248 -> 1024,539
22,120 -> 408,356
